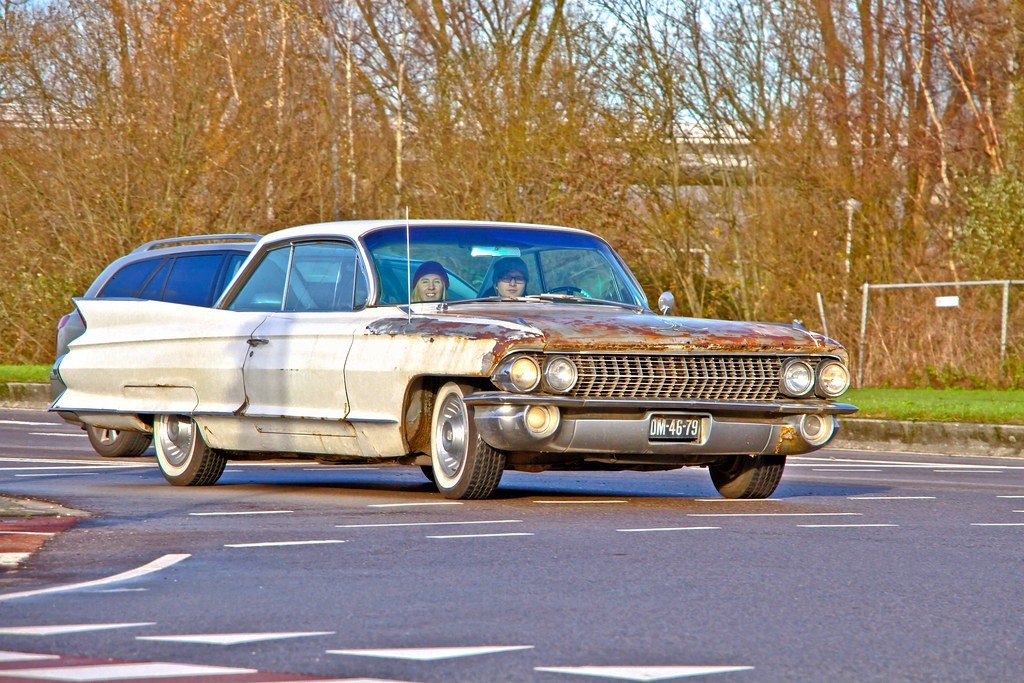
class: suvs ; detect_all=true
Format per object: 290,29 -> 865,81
49,233 -> 479,459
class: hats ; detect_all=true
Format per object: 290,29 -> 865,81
492,257 -> 529,294
413,260 -> 449,290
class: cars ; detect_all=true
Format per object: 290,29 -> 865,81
47,218 -> 860,501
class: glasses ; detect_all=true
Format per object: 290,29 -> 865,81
498,274 -> 527,284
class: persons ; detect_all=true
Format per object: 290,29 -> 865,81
409,260 -> 450,303
494,257 -> 529,299
